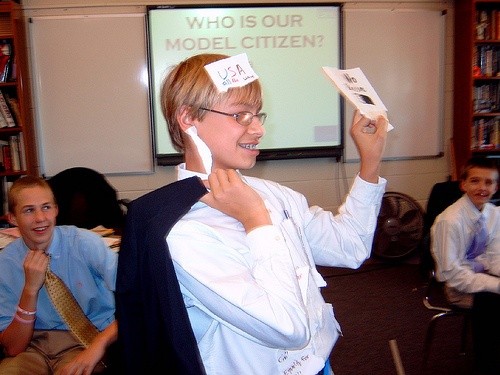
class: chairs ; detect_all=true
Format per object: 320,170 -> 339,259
46,167 -> 132,235
419,180 -> 473,371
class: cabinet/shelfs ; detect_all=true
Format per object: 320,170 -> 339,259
0,0 -> 40,221
452,0 -> 500,180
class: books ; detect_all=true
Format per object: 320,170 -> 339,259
0,90 -> 22,128
471,119 -> 500,149
473,47 -> 500,77
0,133 -> 27,171
473,85 -> 500,113
0,175 -> 27,229
475,10 -> 500,39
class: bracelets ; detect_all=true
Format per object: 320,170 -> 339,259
15,313 -> 36,324
17,304 -> 37,315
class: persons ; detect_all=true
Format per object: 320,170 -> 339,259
429,159 -> 500,375
0,176 -> 118,375
161,54 -> 389,375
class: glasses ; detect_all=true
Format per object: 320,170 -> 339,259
185,103 -> 268,127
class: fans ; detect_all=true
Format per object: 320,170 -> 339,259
371,192 -> 424,260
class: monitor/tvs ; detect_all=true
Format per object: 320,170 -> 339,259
145,1 -> 347,166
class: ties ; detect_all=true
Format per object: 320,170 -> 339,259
465,214 -> 488,271
44,257 -> 99,349
